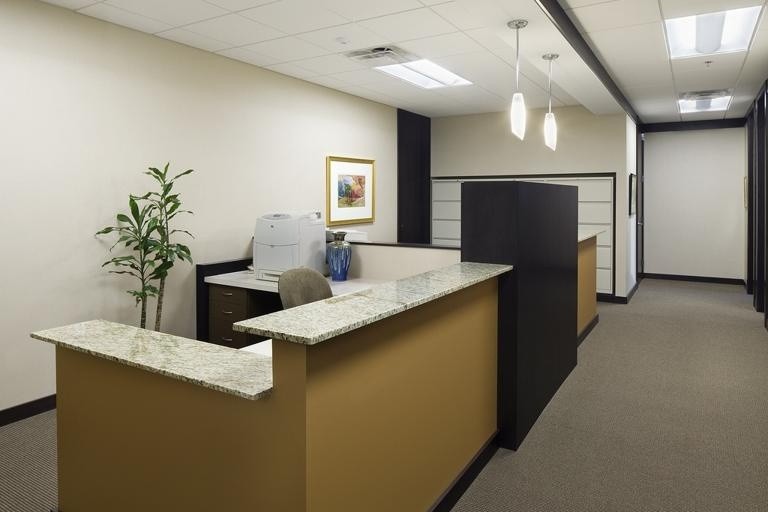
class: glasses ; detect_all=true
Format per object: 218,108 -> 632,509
505,21 -> 561,151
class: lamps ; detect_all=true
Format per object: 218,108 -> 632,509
277,269 -> 334,312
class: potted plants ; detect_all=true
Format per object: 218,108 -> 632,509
325,155 -> 377,226
629,174 -> 637,216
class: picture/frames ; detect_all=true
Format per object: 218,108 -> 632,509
199,268 -> 371,348
